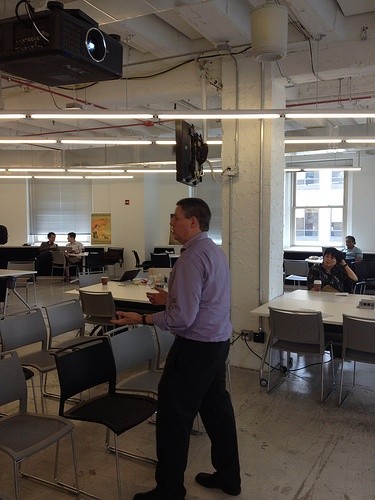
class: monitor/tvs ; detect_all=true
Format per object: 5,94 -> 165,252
174,119 -> 203,187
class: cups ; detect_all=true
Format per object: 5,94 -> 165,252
101,277 -> 109,285
150,276 -> 155,285
314,280 -> 321,291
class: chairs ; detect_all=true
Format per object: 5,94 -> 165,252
0,246 -> 231,500
266,259 -> 375,406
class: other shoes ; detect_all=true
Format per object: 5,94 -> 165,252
27,278 -> 36,282
64,277 -> 70,283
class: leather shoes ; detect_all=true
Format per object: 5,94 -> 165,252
132,489 -> 187,500
195,473 -> 241,495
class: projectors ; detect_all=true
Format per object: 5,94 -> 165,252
0,8 -> 123,86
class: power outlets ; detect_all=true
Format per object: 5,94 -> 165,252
225,165 -> 240,178
253,331 -> 264,343
240,329 -> 252,341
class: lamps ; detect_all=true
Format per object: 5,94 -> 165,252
0,45 -> 375,179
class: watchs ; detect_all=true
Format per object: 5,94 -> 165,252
142,313 -> 147,324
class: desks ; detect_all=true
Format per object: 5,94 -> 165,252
0,253 -> 375,388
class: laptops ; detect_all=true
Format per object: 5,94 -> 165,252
111,269 -> 141,282
58,247 -> 72,254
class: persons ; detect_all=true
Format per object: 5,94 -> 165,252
307,248 -> 358,293
110,197 -> 241,500
65,233 -> 84,264
340,236 -> 363,278
27,232 -> 58,282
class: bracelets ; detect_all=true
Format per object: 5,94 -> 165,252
343,263 -> 348,267
